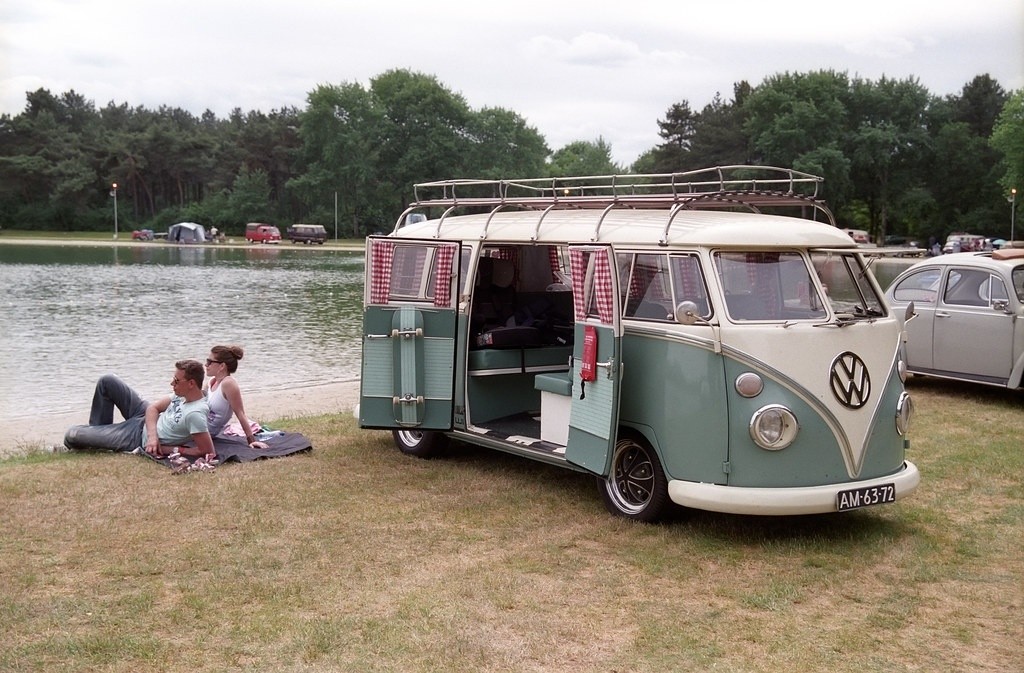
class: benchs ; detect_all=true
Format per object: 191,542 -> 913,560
533,365 -> 573,448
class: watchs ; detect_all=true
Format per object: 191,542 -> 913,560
173,446 -> 178,454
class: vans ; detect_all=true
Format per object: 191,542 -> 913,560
288,224 -> 328,244
351,162 -> 926,523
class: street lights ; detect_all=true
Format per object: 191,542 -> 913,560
1010,188 -> 1016,243
112,183 -> 119,239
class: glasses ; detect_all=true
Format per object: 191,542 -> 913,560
173,375 -> 192,384
207,358 -> 223,366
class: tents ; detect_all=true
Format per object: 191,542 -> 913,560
170,221 -> 206,242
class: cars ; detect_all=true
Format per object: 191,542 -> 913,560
865,248 -> 1024,400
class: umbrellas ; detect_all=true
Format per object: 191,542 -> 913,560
992,239 -> 1007,246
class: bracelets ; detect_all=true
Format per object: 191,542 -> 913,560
176,446 -> 185,455
247,435 -> 255,444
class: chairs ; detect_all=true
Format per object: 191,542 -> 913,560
700,293 -> 766,321
423,258 -> 573,377
623,299 -> 668,318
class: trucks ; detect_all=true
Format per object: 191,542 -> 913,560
246,222 -> 282,244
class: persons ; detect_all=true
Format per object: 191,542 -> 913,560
64,359 -> 215,458
960,238 -> 986,252
201,346 -> 270,450
210,226 -> 219,243
